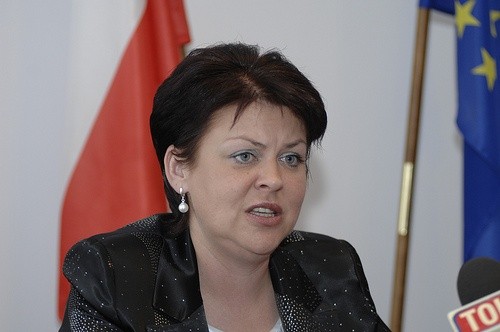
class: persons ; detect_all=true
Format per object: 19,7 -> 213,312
58,43 -> 391,332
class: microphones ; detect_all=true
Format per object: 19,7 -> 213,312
448,258 -> 500,332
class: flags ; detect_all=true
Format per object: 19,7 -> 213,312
58,0 -> 191,319
420,1 -> 500,263
